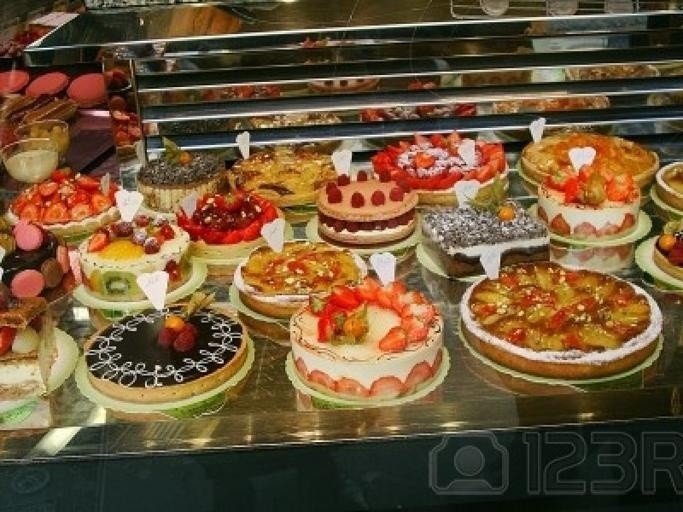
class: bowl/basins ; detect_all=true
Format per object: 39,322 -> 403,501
13,119 -> 69,159
0,137 -> 61,185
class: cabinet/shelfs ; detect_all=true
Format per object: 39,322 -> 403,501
1,157 -> 679,453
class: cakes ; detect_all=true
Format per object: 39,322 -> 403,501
537,161 -> 640,240
80,302 -> 246,399
316,170 -> 418,248
234,240 -> 370,318
137,150 -> 226,210
205,84 -> 286,102
422,203 -> 550,278
73,217 -> 193,302
491,96 -> 608,112
238,113 -> 343,129
460,261 -> 663,380
363,104 -> 480,123
0,209 -> 68,302
654,217 -> 683,280
655,161 -> 683,214
372,132 -> 510,206
0,304 -> 58,397
289,278 -> 444,399
107,100 -> 153,153
521,133 -> 659,181
3,167 -> 127,236
646,93 -> 683,134
306,76 -> 382,93
565,65 -> 661,85
177,189 -> 285,259
233,149 -> 338,208
